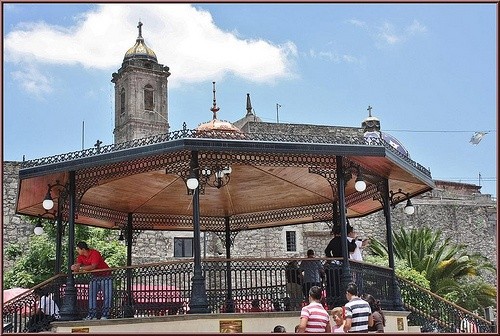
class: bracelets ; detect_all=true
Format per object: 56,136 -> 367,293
80,267 -> 82,269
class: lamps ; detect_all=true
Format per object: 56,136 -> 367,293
203,164 -> 232,190
33,210 -> 56,235
345,165 -> 366,192
185,171 -> 198,189
42,180 -> 67,210
390,188 -> 415,215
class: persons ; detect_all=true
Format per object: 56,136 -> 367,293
20,288 -> 61,333
459,315 -> 477,333
71,241 -> 112,320
286,224 -> 384,333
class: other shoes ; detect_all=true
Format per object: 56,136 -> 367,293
85,316 -> 97,320
100,316 -> 108,320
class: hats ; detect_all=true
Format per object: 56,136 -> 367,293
346,224 -> 353,235
